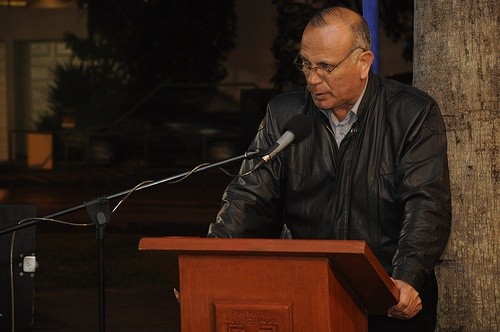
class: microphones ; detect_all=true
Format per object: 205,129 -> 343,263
254,114 -> 312,171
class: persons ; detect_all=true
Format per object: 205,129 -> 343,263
204,5 -> 452,332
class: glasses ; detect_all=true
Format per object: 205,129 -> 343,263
293,49 -> 357,75
